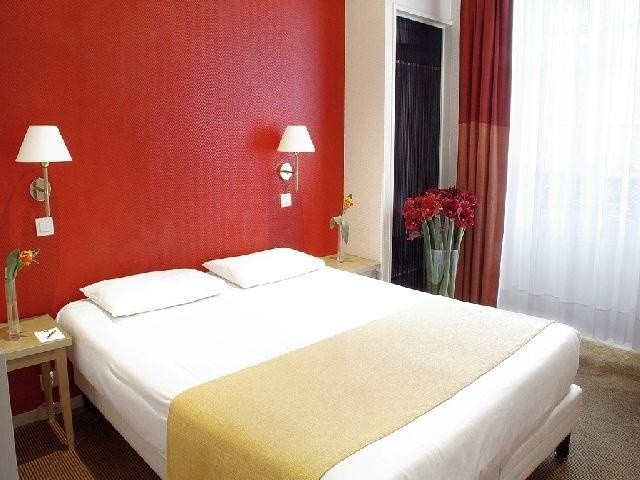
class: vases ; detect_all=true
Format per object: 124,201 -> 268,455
5,279 -> 20,340
337,228 -> 345,262
425,248 -> 459,298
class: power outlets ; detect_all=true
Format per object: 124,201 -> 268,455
280,193 -> 291,208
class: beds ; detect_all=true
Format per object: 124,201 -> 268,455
55,271 -> 583,480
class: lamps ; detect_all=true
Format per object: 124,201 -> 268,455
277,125 -> 315,193
15,125 -> 72,216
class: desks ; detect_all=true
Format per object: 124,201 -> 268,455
0,315 -> 76,451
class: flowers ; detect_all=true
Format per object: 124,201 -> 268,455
402,187 -> 479,295
5,248 -> 39,318
329,194 -> 354,245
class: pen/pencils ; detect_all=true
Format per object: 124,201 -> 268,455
46,330 -> 56,338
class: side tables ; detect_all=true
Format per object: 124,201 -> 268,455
319,252 -> 378,279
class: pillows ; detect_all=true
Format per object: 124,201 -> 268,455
80,269 -> 230,318
202,247 -> 325,289
